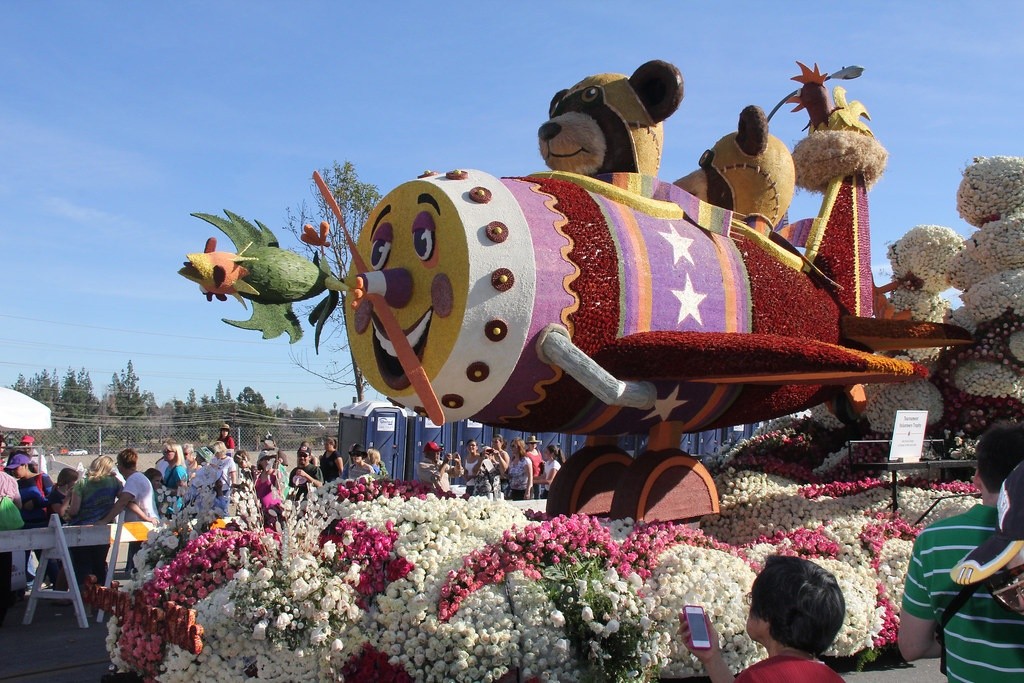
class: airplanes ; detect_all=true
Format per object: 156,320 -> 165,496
177,62 -> 973,528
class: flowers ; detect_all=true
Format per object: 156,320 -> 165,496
866,154 -> 1024,437
105,427 -> 984,683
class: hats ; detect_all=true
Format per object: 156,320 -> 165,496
258,450 -> 277,462
20,435 -> 34,443
297,446 -> 311,456
219,424 -> 231,432
525,436 -> 540,443
4,454 -> 31,472
348,444 -> 368,459
262,440 -> 279,450
950,462 -> 1024,585
424,441 -> 444,452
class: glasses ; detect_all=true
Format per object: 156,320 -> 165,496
990,574 -> 1024,616
510,444 -> 517,447
298,453 -> 309,457
115,462 -> 118,466
351,452 -> 363,456
163,450 -> 174,454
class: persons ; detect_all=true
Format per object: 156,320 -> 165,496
898,422 -> 1024,683
0,425 -> 381,618
679,555 -> 847,683
464,434 -> 566,502
417,442 -> 463,493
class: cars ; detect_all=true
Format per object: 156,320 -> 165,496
68,449 -> 87,456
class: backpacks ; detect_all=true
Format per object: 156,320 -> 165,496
0,496 -> 25,531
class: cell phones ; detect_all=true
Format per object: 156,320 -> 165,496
682,605 -> 711,650
485,449 -> 493,453
451,454 -> 458,459
